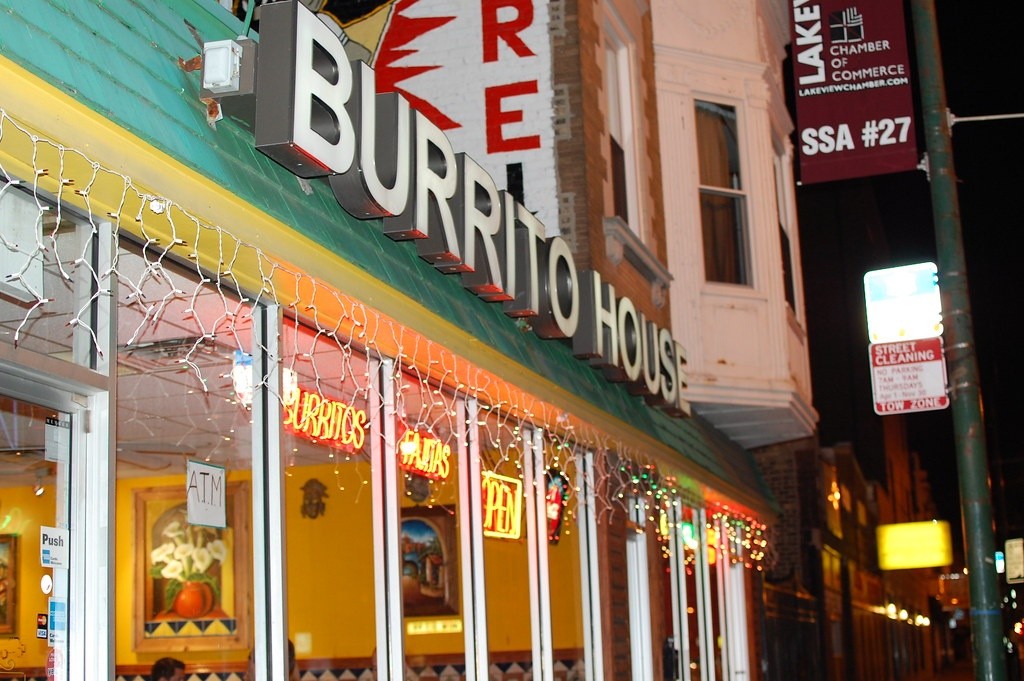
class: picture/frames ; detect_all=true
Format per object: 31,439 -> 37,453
0,533 -> 18,635
130,480 -> 250,653
400,504 -> 459,617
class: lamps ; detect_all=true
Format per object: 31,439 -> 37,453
202,38 -> 243,94
31,476 -> 44,496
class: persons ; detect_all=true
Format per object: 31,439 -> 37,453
151,657 -> 185,681
